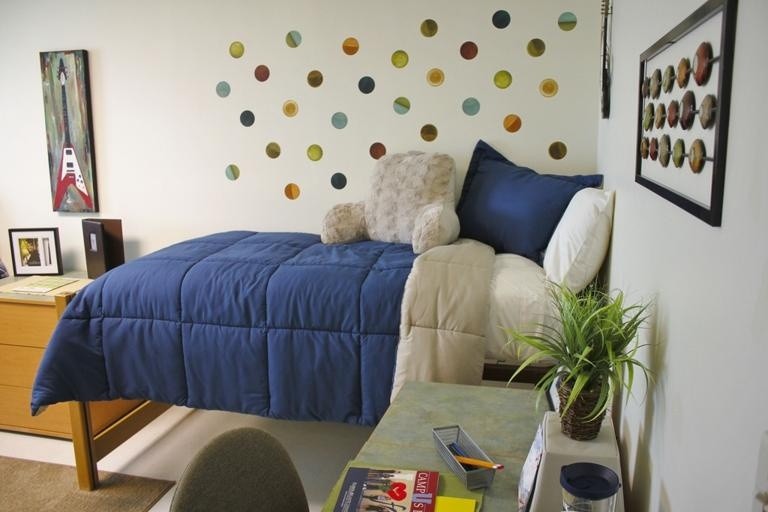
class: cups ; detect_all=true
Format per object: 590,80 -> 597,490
560,462 -> 621,512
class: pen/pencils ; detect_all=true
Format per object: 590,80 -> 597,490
446,443 -> 505,471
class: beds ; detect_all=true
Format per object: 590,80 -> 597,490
22,224 -> 604,495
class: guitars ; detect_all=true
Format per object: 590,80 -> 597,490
600,1 -> 612,119
53,59 -> 93,213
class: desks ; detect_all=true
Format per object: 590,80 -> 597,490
318,375 -> 632,512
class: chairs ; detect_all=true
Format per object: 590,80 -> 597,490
166,423 -> 316,510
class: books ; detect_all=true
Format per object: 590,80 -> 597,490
11,276 -> 79,293
434,495 -> 475,512
334,463 -> 439,512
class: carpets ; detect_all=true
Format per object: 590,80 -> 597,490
1,448 -> 180,511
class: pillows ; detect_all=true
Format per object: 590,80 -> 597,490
540,185 -> 618,296
449,137 -> 603,270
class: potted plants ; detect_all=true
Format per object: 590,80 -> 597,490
496,276 -> 668,446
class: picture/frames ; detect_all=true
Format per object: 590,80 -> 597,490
6,225 -> 65,277
633,1 -> 744,231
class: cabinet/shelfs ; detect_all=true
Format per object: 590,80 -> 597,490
1,266 -> 185,436
